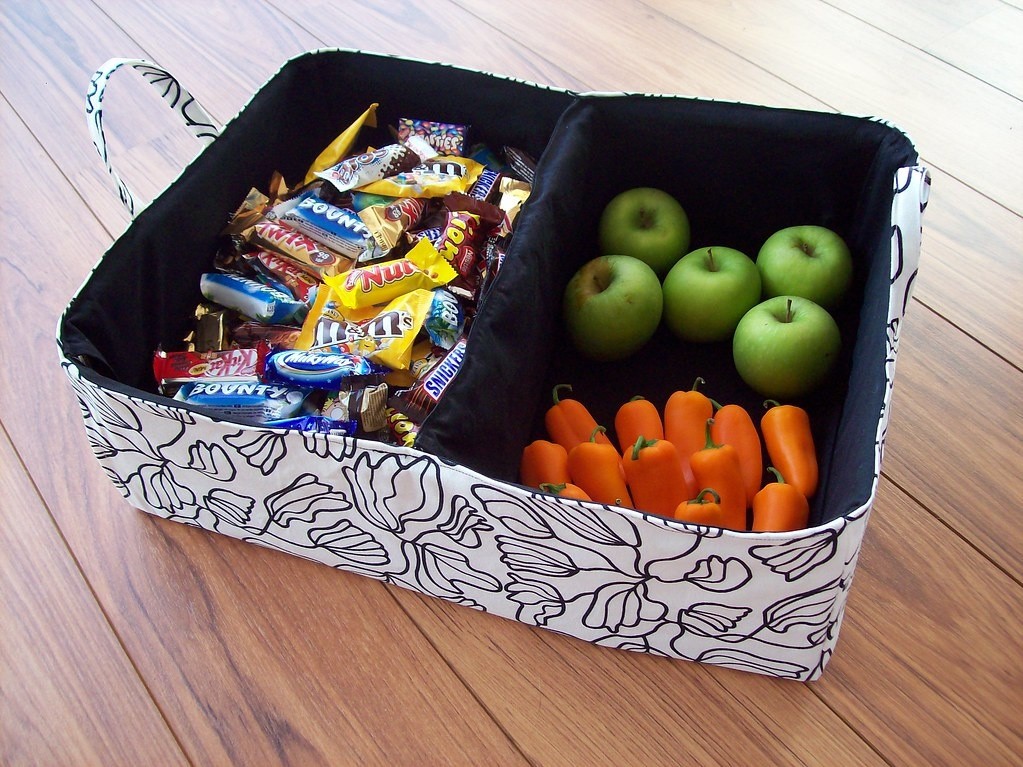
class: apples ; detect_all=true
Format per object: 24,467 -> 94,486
733,295 -> 840,400
662,246 -> 760,345
563,254 -> 663,359
757,225 -> 850,308
598,187 -> 689,280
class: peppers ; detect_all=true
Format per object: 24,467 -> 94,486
521,376 -> 817,534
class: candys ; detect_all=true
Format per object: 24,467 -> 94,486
149,102 -> 536,449
